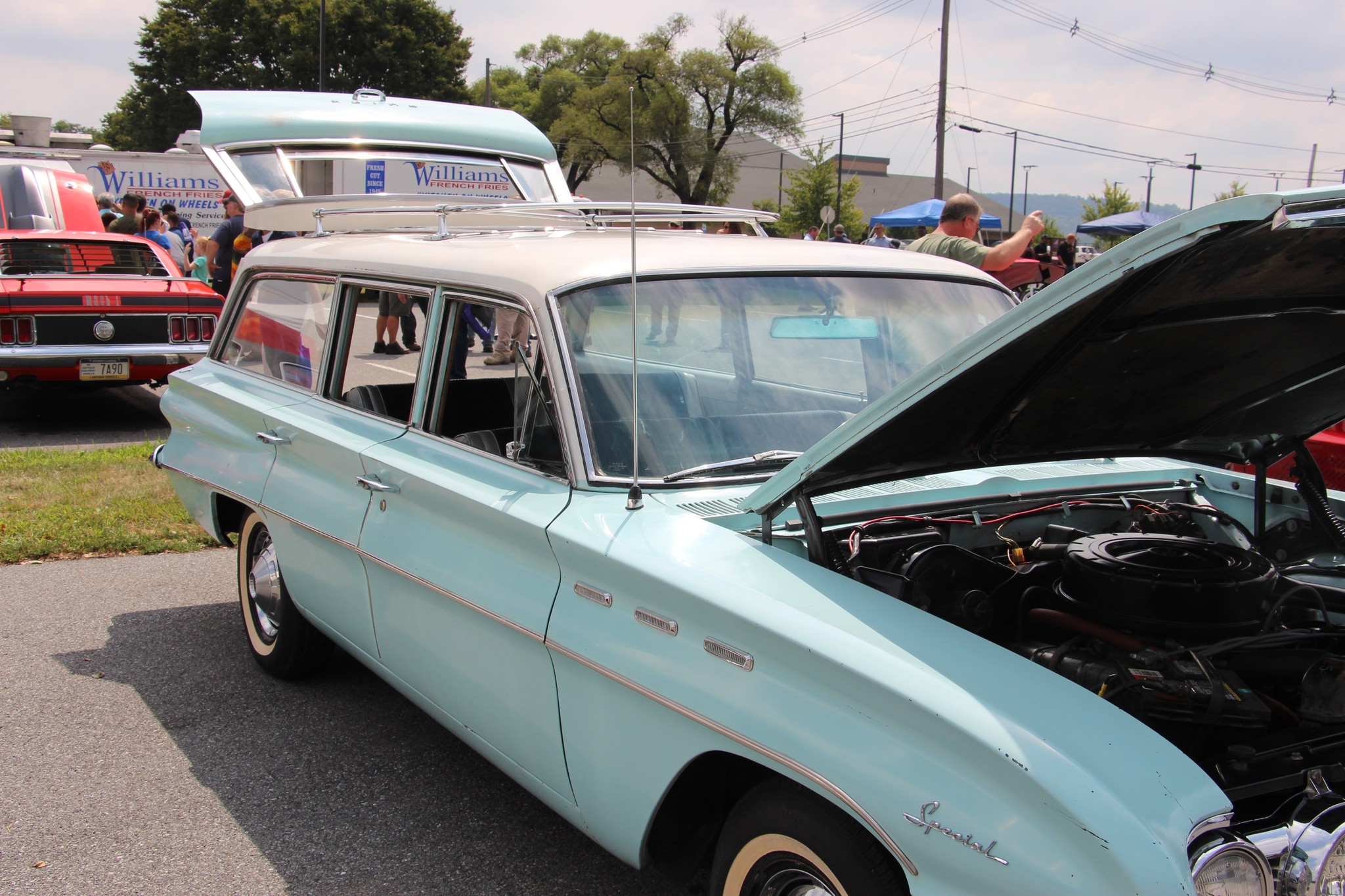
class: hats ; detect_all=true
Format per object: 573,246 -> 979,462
222,194 -> 237,202
1041,234 -> 1051,239
669,221 -> 683,227
834,224 -> 844,232
889,239 -> 900,249
1067,233 -> 1078,240
216,189 -> 232,203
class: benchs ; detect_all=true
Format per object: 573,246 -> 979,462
452,409 -> 855,489
342,370 -> 703,439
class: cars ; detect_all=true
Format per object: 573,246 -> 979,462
141,89 -> 1345,896
1223,413 -> 1345,492
0,162 -> 224,397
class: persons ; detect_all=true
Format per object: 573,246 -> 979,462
98,192 -> 209,284
867,223 -> 891,248
1022,234 -> 1052,262
888,237 -> 900,249
826,224 -> 851,243
715,222 -> 741,350
645,220 -> 684,344
798,225 -> 820,311
904,193 -> 1045,271
204,184 -> 330,388
372,289 -> 532,365
1056,231 -> 1076,274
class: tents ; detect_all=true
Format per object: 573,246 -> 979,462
1074,209 -> 1169,250
863,197 -> 1001,247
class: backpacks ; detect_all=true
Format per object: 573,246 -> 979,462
179,222 -> 193,244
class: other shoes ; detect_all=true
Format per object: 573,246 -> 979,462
385,341 -> 410,355
406,342 -> 421,351
483,345 -> 494,353
483,354 -> 510,365
468,341 -> 475,348
373,340 -> 387,353
646,330 -> 662,340
509,348 -> 528,362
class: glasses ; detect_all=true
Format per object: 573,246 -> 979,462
874,227 -> 882,230
723,225 -> 730,229
670,223 -> 679,228
963,216 -> 981,233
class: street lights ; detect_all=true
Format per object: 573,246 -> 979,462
779,152 -> 791,214
1114,181 -> 1124,203
967,167 -> 979,194
1023,165 -> 1038,216
832,113 -> 844,224
1185,153 -> 1197,211
